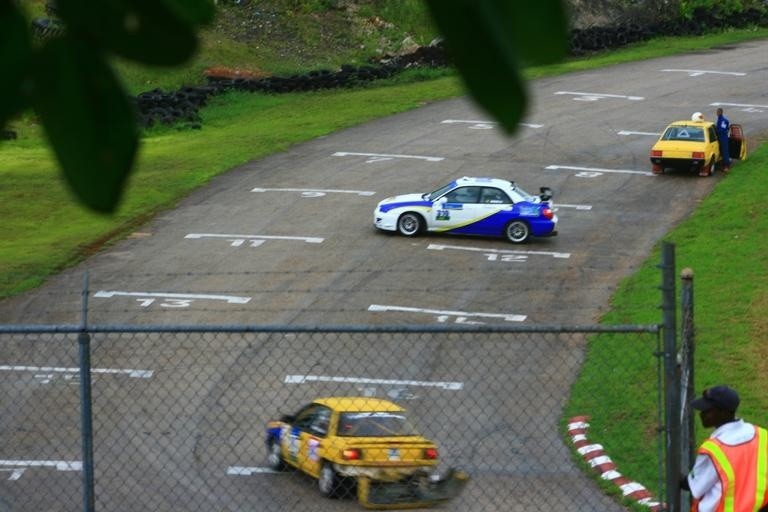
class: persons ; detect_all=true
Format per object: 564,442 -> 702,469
716,108 -> 732,172
681,385 -> 768,511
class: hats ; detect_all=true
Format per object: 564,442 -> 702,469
691,386 -> 739,411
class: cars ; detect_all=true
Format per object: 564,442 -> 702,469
265,396 -> 469,509
650,118 -> 748,177
373,175 -> 558,244
